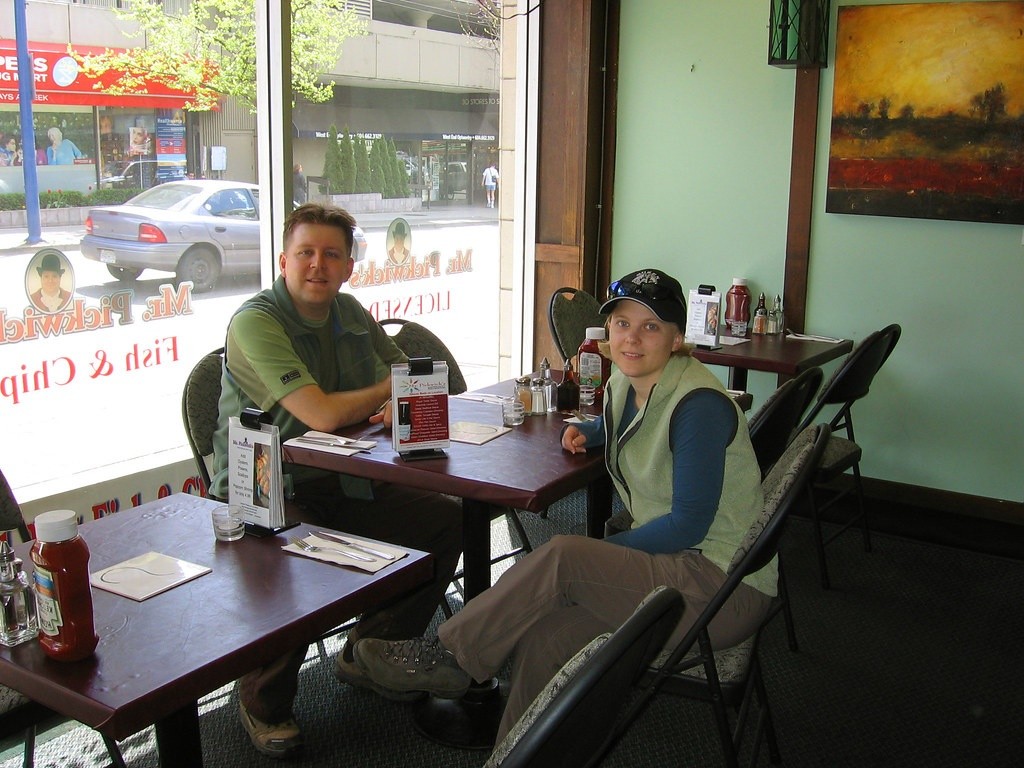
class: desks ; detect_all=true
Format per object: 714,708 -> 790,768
284,370 -> 754,751
0,492 -> 432,768
690,325 -> 853,391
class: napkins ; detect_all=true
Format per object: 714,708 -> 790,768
785,334 -> 845,343
284,430 -> 375,457
282,532 -> 408,574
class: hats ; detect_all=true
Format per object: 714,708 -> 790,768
36,254 -> 65,277
599,269 -> 686,336
392,222 -> 407,239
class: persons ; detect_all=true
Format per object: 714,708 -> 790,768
0,132 -> 17,166
482,162 -> 499,208
46,127 -> 82,165
293,163 -> 307,202
207,202 -> 463,757
132,127 -> 147,154
13,135 -> 48,166
389,223 -> 409,264
30,254 -> 71,312
354,269 -> 779,754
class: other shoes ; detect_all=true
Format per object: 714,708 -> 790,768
491,204 -> 494,208
487,204 -> 491,207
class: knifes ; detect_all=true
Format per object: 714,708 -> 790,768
309,531 -> 394,559
297,439 -> 370,450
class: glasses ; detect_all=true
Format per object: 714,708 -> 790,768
606,281 -> 686,309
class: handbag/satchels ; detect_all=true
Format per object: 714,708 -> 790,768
492,177 -> 497,182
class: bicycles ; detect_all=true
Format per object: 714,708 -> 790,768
438,174 -> 455,201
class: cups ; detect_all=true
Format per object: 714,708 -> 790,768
502,401 -> 525,425
579,385 -> 595,405
732,320 -> 748,337
212,506 -> 244,541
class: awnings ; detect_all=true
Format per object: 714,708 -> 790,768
0,38 -> 224,112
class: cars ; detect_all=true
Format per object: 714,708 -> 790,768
99,160 -> 158,190
81,178 -> 367,295
395,150 -> 470,201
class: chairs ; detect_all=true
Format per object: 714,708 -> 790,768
577,422 -> 831,768
0,471 -> 131,768
482,584 -> 683,768
792,323 -> 902,589
548,287 -> 603,368
181,348 -> 359,641
744,367 -> 824,478
377,318 -> 533,621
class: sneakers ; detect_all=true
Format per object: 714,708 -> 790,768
353,637 -> 471,698
238,702 -> 301,758
331,627 -> 425,702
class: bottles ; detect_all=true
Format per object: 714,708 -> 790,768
514,376 -> 532,416
557,359 -> 579,414
773,295 -> 783,333
30,510 -> 99,661
754,309 -> 766,334
768,310 -> 778,335
577,327 -> 612,399
754,292 -> 767,318
0,531 -> 37,645
725,278 -> 751,328
531,378 -> 547,416
540,358 -> 558,411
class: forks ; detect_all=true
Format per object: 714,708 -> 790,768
300,435 -> 366,444
288,536 -> 375,563
